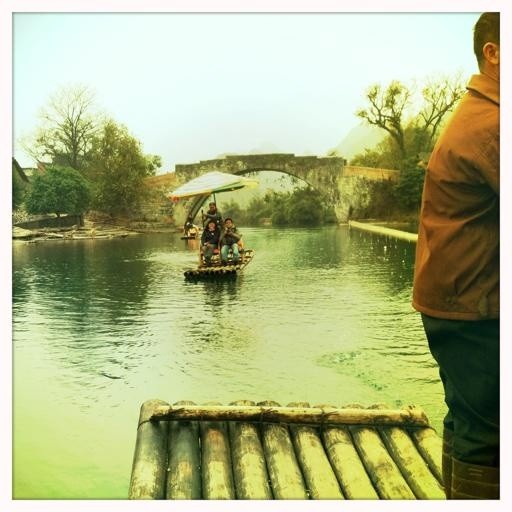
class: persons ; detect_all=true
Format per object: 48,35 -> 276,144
218,218 -> 242,264
199,220 -> 220,266
201,201 -> 224,224
184,211 -> 199,239
408,14 -> 499,499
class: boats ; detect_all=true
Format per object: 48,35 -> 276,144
183,247 -> 255,278
180,226 -> 198,239
125,399 -> 447,499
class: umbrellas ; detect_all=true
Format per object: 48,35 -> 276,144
164,171 -> 258,203
